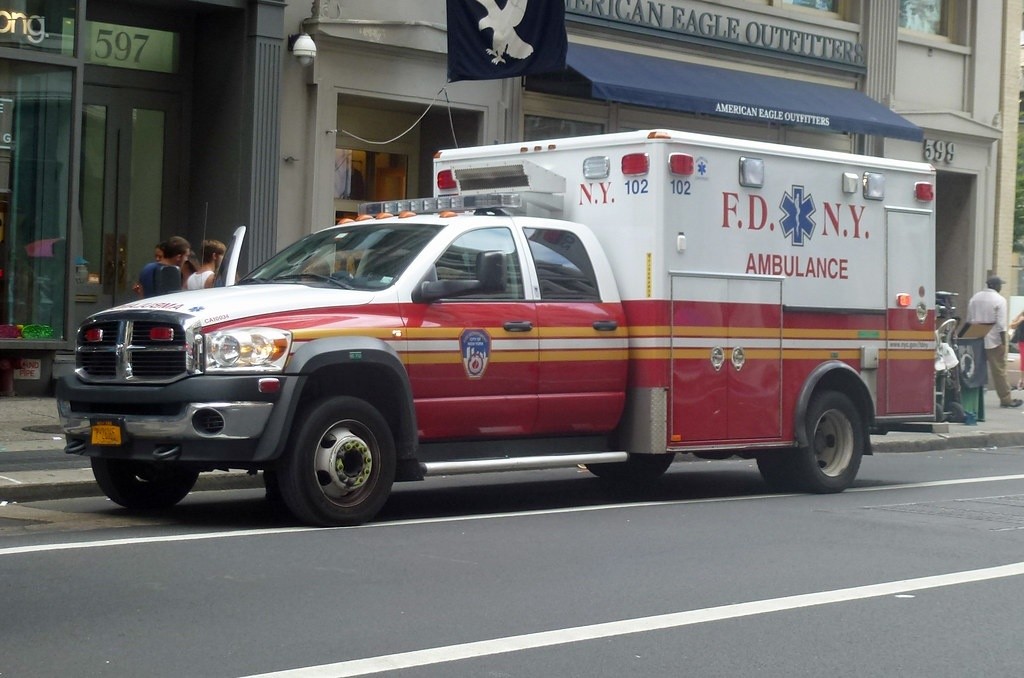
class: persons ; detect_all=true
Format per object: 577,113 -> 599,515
968,277 -> 1024,408
133,237 -> 226,299
1010,311 -> 1024,390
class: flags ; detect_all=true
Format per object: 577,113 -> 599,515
445,0 -> 568,83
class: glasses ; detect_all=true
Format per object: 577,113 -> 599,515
184,260 -> 189,264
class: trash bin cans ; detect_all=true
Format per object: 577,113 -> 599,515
946,336 -> 986,423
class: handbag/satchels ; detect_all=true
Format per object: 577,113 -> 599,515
1010,327 -> 1020,343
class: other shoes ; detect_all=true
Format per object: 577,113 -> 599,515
1000,399 -> 1023,407
1018,379 -> 1024,390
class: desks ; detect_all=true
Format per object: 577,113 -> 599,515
0,338 -> 70,398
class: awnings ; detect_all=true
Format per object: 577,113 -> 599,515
525,42 -> 924,142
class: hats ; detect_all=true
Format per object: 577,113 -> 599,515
985,276 -> 1006,285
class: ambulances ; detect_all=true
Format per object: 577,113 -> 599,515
52,127 -> 938,523
